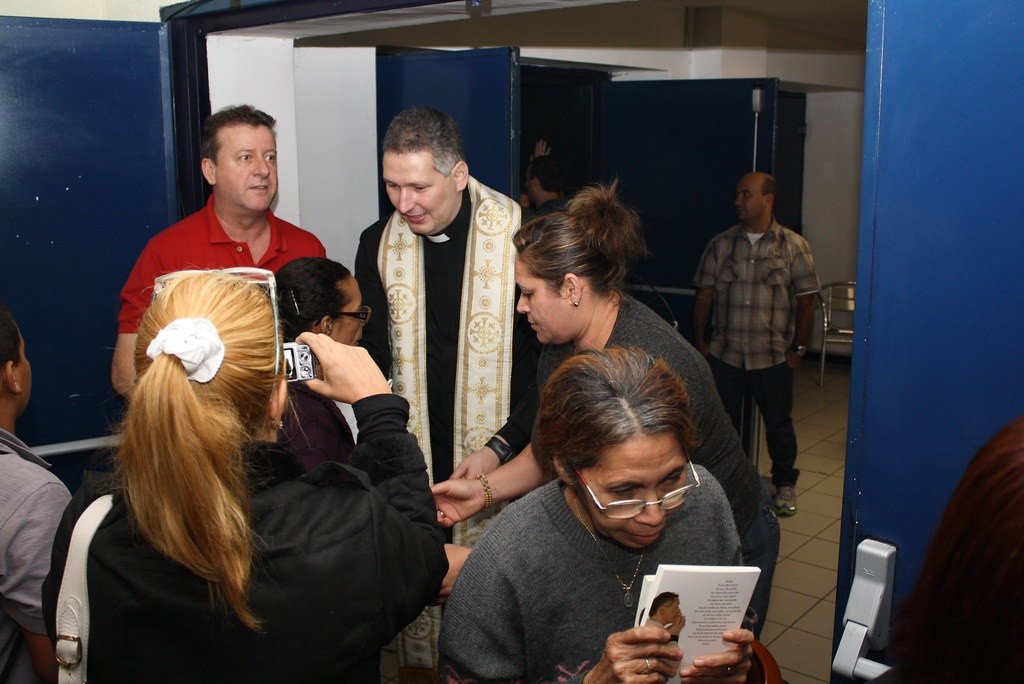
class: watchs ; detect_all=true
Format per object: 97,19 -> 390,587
791,343 -> 808,357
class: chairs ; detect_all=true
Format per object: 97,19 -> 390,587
817,281 -> 857,387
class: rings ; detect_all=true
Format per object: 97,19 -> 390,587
441,511 -> 444,518
728,667 -> 731,670
645,659 -> 651,669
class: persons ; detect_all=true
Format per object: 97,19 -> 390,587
111,105 -> 327,399
436,345 -> 759,684
355,106 -> 544,544
519,139 -> 577,224
430,186 -> 780,640
276,256 -> 373,474
691,171 -> 821,516
645,592 -> 686,672
866,418 -> 1024,684
0,300 -> 72,684
41,266 -> 450,684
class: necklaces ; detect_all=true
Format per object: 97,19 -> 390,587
574,496 -> 644,607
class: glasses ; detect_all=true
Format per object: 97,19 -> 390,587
315,305 -> 371,328
568,446 -> 701,519
152,266 -> 280,402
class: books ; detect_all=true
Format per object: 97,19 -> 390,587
633,563 -> 761,684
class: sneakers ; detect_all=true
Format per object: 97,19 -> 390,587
773,485 -> 798,516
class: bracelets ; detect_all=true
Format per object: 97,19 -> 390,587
475,474 -> 492,508
484,436 -> 516,464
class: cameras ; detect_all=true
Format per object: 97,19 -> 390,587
283,341 -> 317,383
